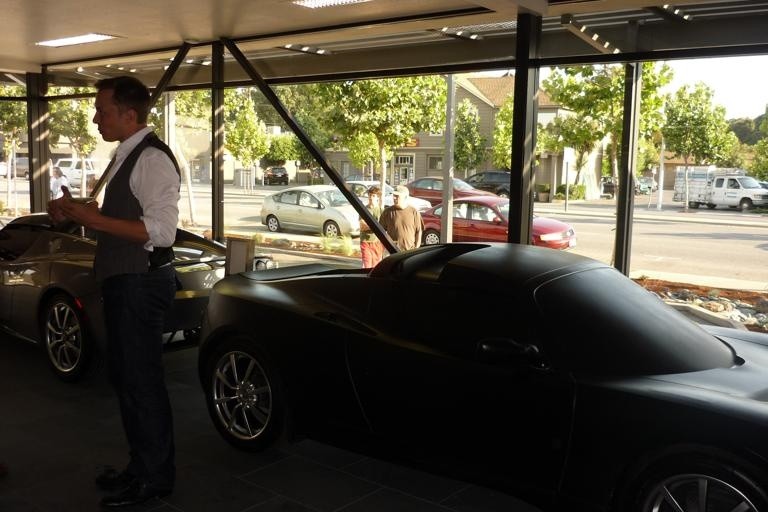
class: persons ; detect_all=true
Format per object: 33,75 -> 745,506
48,76 -> 181,507
53,167 -> 70,199
359,187 -> 384,268
379,185 -> 425,259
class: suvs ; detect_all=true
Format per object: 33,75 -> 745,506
54,158 -> 95,189
264,167 -> 289,185
463,171 -> 510,198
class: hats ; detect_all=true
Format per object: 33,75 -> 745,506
390,186 -> 409,196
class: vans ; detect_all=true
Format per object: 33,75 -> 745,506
4,157 -> 54,180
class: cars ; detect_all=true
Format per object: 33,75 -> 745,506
343,180 -> 433,210
601,176 -> 658,198
419,195 -> 578,251
403,177 -> 499,207
260,184 -> 361,237
758,182 -> 768,189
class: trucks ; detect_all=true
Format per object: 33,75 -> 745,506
672,165 -> 768,211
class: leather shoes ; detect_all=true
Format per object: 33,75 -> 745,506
101,476 -> 174,511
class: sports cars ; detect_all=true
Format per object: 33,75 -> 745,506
0,214 -> 278,376
196,241 -> 768,512
